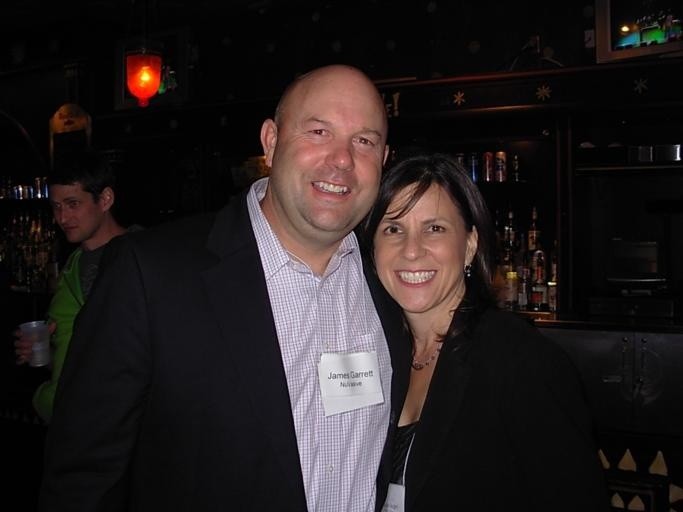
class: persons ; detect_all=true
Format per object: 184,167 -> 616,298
361,151 -> 616,512
13,156 -> 146,511
34,61 -> 410,512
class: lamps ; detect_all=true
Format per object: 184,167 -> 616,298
123,30 -> 163,108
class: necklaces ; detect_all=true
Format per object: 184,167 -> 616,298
411,340 -> 445,371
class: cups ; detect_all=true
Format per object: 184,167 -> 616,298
18,319 -> 49,367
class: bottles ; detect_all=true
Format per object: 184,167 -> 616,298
0,213 -> 56,291
489,205 -> 556,313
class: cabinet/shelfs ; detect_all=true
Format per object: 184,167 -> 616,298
462,158 -> 557,314
537,324 -> 682,511
